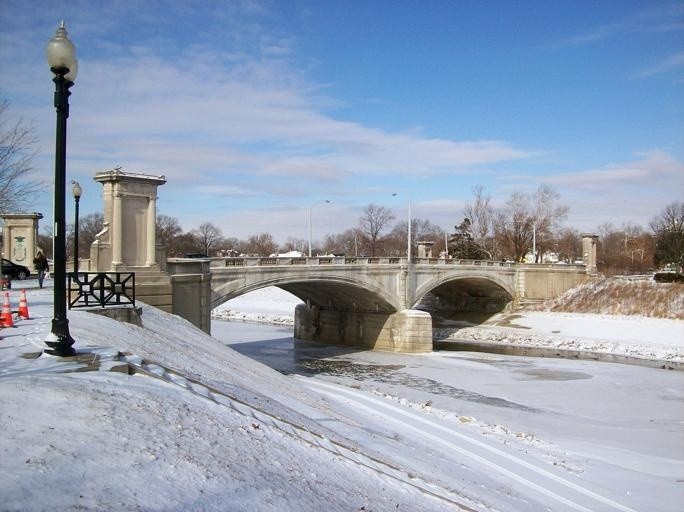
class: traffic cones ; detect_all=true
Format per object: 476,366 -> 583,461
0,288 -> 30,328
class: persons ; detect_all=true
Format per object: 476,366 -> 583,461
33,251 -> 49,289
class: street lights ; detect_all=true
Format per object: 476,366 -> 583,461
43,20 -> 82,356
392,191 -> 412,263
515,221 -> 536,264
308,199 -> 330,257
70,180 -> 82,283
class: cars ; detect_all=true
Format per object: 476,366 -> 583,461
0,258 -> 30,280
